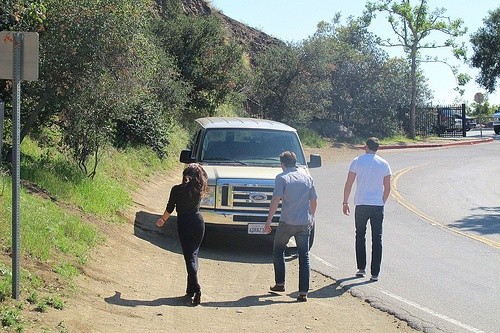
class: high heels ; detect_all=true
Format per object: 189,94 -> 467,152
184,291 -> 201,305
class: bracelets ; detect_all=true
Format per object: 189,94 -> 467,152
160,218 -> 165,223
343,203 -> 348,205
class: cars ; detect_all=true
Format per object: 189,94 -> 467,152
179,116 -> 323,251
493,106 -> 500,135
435,111 -> 477,133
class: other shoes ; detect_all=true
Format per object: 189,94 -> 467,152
370,275 -> 378,280
355,269 -> 366,274
297,294 -> 307,301
270,285 -> 285,292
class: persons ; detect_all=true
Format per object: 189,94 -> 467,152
264,151 -> 317,301
342,137 -> 392,280
156,163 -> 208,305
